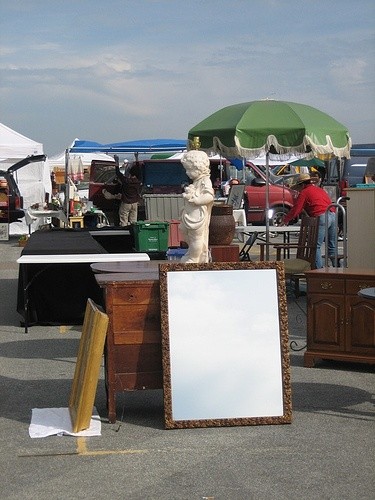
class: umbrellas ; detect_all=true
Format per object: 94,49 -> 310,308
188,99 -> 352,261
288,157 -> 326,167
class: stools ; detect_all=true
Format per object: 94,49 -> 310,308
69,216 -> 85,228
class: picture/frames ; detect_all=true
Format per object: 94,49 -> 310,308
159,261 -> 293,428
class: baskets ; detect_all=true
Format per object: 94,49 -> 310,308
18,235 -> 29,247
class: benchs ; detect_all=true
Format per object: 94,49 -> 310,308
256,243 -> 298,261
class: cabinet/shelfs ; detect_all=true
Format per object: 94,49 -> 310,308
94,272 -> 170,425
303,267 -> 375,368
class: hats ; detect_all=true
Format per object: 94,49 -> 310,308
290,174 -> 319,191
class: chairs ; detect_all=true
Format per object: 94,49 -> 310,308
284,216 -> 320,299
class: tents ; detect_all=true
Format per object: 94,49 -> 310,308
65,138 -> 246,227
49,137 -> 115,172
0,123 -> 52,234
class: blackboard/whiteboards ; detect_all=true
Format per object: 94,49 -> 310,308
226,183 -> 245,210
158,260 -> 294,430
344,188 -> 375,268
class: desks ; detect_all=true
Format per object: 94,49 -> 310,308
25,210 -> 69,234
236,226 -> 300,259
16,253 -> 150,333
232,208 -> 247,243
90,260 -> 167,273
210,245 -> 239,262
16,228 -> 108,327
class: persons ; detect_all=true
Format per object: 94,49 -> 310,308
115,167 -> 143,227
276,172 -> 339,269
51,172 -> 58,197
180,150 -> 215,264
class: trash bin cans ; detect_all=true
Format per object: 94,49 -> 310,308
145,218 -> 183,248
127,219 -> 169,260
